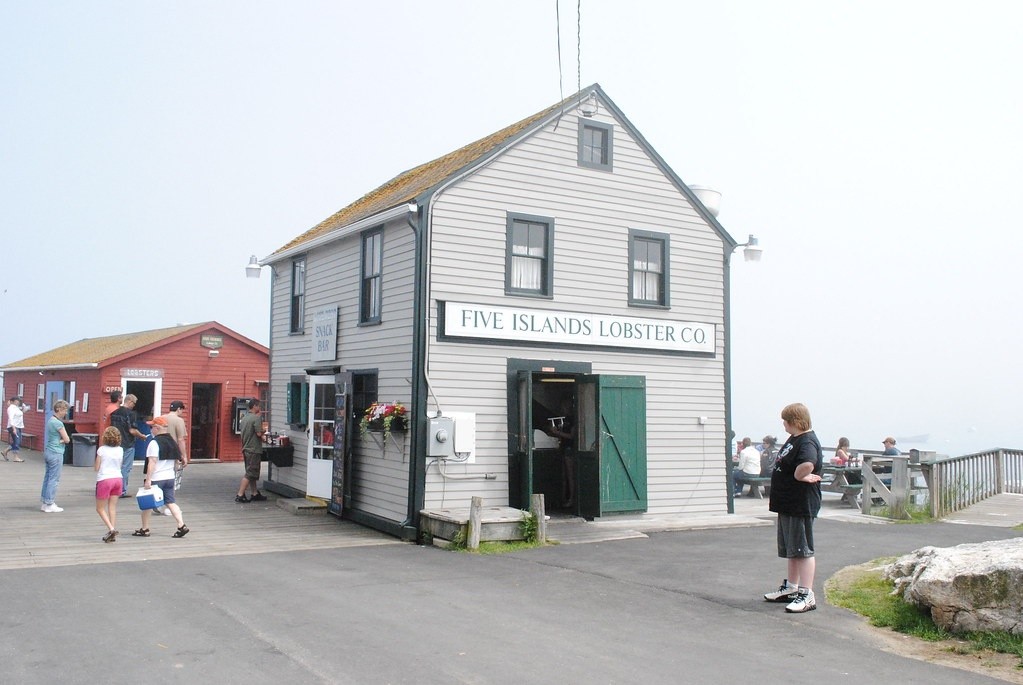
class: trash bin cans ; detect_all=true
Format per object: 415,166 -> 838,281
71,433 -> 98,467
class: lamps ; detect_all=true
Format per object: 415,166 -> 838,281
208,350 -> 219,358
726,235 -> 762,266
246,255 -> 279,279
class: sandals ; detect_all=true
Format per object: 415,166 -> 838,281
132,527 -> 150,537
172,524 -> 189,538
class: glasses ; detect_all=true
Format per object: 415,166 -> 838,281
763,442 -> 769,446
150,425 -> 158,429
131,401 -> 137,406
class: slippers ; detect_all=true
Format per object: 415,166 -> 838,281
13,458 -> 25,462
1,451 -> 9,461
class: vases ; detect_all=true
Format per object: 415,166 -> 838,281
370,417 -> 403,430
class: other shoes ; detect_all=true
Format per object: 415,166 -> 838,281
735,491 -> 742,497
164,508 -> 183,516
102,529 -> 119,543
153,508 -> 161,513
747,492 -> 755,497
873,500 -> 886,505
119,495 -> 132,498
840,498 -> 851,505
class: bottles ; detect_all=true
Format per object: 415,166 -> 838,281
264,430 -> 289,446
849,453 -> 858,467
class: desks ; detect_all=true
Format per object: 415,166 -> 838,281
733,462 -> 771,499
820,467 -> 885,509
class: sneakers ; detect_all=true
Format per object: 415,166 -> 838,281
763,579 -> 798,603
785,586 -> 816,612
251,490 -> 267,501
235,493 -> 251,503
40,503 -> 63,513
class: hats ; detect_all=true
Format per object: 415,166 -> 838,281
170,400 -> 185,409
882,436 -> 895,445
146,416 -> 169,427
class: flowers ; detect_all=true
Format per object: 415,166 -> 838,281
359,400 -> 408,447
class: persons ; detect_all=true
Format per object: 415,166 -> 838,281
133,417 -> 189,538
103,391 -> 123,429
733,437 -> 761,498
835,436 -> 862,484
1,397 -> 25,462
763,403 -> 824,612
154,400 -> 188,514
761,435 -> 781,477
874,437 -> 901,505
39,400 -> 71,512
550,412 -> 577,507
94,426 -> 124,543
109,394 -> 146,498
234,398 -> 267,502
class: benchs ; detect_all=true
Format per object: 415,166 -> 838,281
4,430 -> 36,450
746,478 -> 771,482
840,484 -> 891,489
